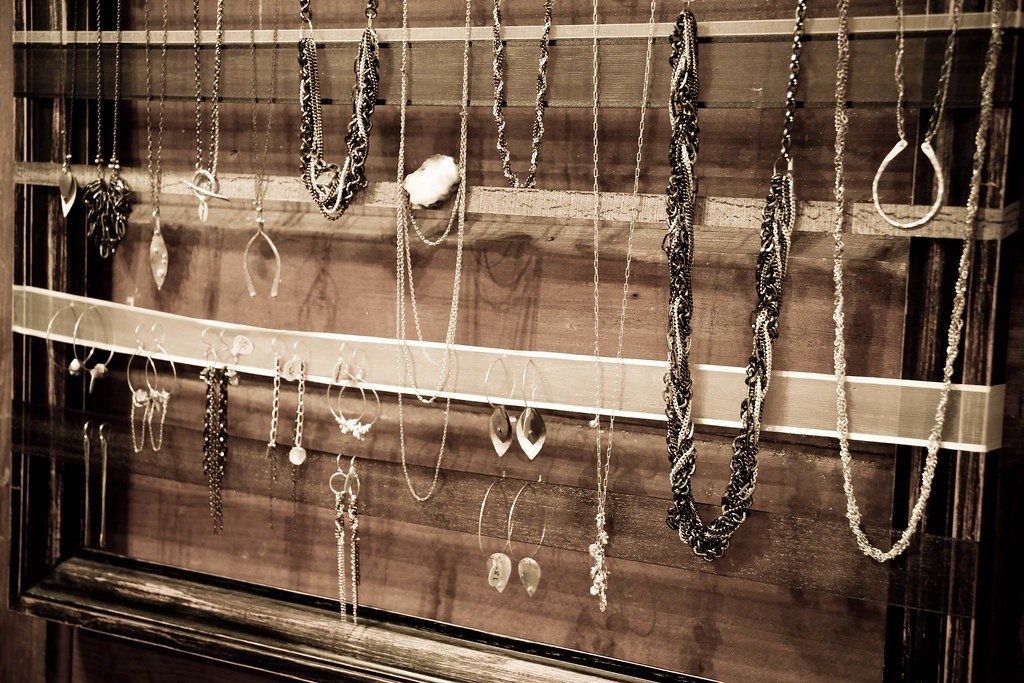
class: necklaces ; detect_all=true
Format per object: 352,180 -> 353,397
144,0 -> 169,291
836,0 -> 1007,565
56,0 -> 77,217
662,0 -> 807,563
490,0 -> 552,189
179,0 -> 230,223
82,0 -> 133,260
298,0 -> 381,218
242,0 -> 282,300
395,1 -> 471,501
588,0 -> 656,612
